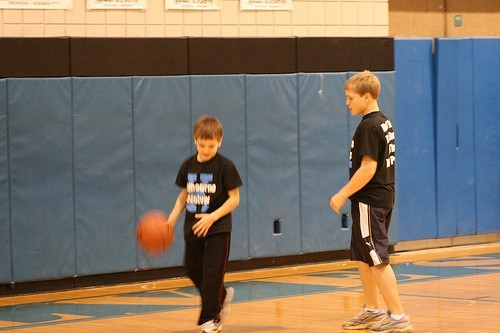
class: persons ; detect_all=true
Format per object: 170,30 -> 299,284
329,70 -> 414,332
167,116 -> 244,333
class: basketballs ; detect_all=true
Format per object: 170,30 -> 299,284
134,209 -> 174,255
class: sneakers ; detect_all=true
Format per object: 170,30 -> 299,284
200,319 -> 223,333
342,309 -> 385,329
224,286 -> 234,307
371,310 -> 414,333
